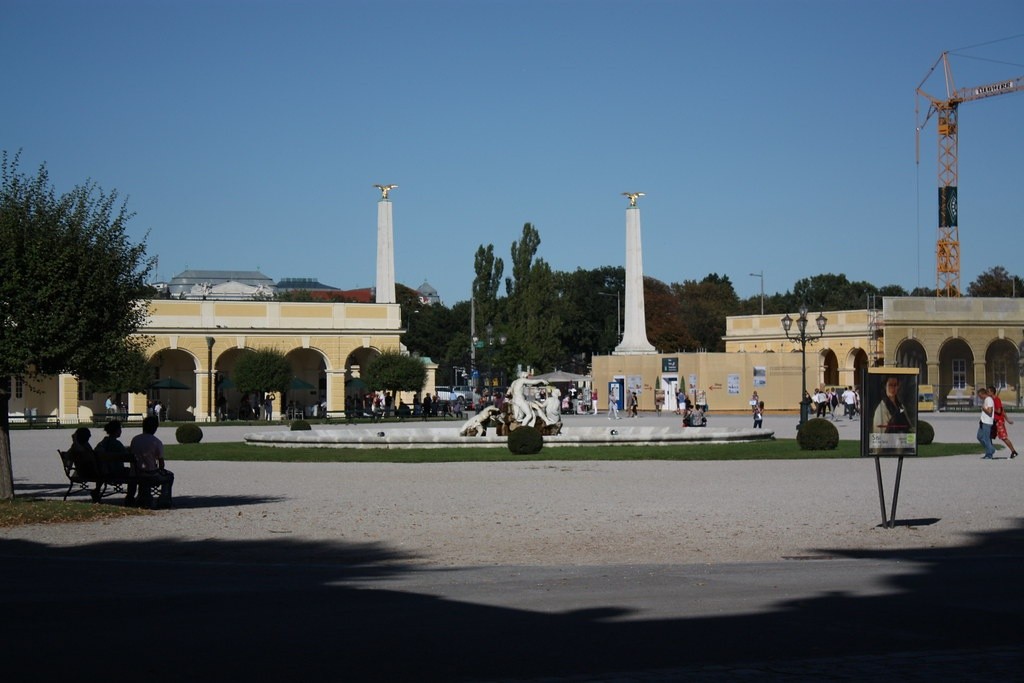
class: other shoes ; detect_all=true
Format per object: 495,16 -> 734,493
147,496 -> 154,502
124,496 -> 135,506
91,490 -> 101,502
158,496 -> 170,505
135,499 -> 146,507
1011,450 -> 1018,458
982,454 -> 992,460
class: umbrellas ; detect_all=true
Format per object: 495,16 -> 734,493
150,375 -> 190,420
345,378 -> 369,390
286,377 -> 316,400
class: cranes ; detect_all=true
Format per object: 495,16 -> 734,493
916,33 -> 1024,298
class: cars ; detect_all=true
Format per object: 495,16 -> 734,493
434,383 -> 508,403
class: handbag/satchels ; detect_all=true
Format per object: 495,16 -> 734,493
683,415 -> 691,426
749,400 -> 757,406
755,413 -> 761,421
806,396 -> 812,405
990,420 -> 997,439
702,418 -> 707,422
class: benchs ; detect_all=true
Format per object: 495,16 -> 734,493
8,415 -> 60,430
321,404 -> 469,425
58,447 -> 163,504
945,399 -> 973,411
104,408 -> 127,421
90,414 -> 144,428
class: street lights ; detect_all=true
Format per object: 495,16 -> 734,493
749,271 -> 763,314
780,302 -> 828,430
472,322 -> 508,403
597,289 -> 620,345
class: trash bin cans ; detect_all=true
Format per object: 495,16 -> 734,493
24,408 -> 37,422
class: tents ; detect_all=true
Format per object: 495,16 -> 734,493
537,370 -> 595,401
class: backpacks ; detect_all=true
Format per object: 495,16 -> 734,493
831,394 -> 838,408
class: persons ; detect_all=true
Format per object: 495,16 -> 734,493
473,388 -> 501,422
147,398 -> 167,422
504,370 -> 562,428
975,386 -> 1019,459
346,392 -> 391,418
563,385 -> 639,419
106,395 -> 117,413
264,393 -> 276,421
68,427 -> 103,501
677,383 -> 709,426
805,385 -> 861,421
287,400 -> 327,419
242,391 -> 250,405
412,392 -> 462,421
130,414 -> 174,506
252,392 -> 260,420
871,373 -> 915,454
93,418 -> 136,504
750,390 -> 765,428
218,392 -> 227,421
